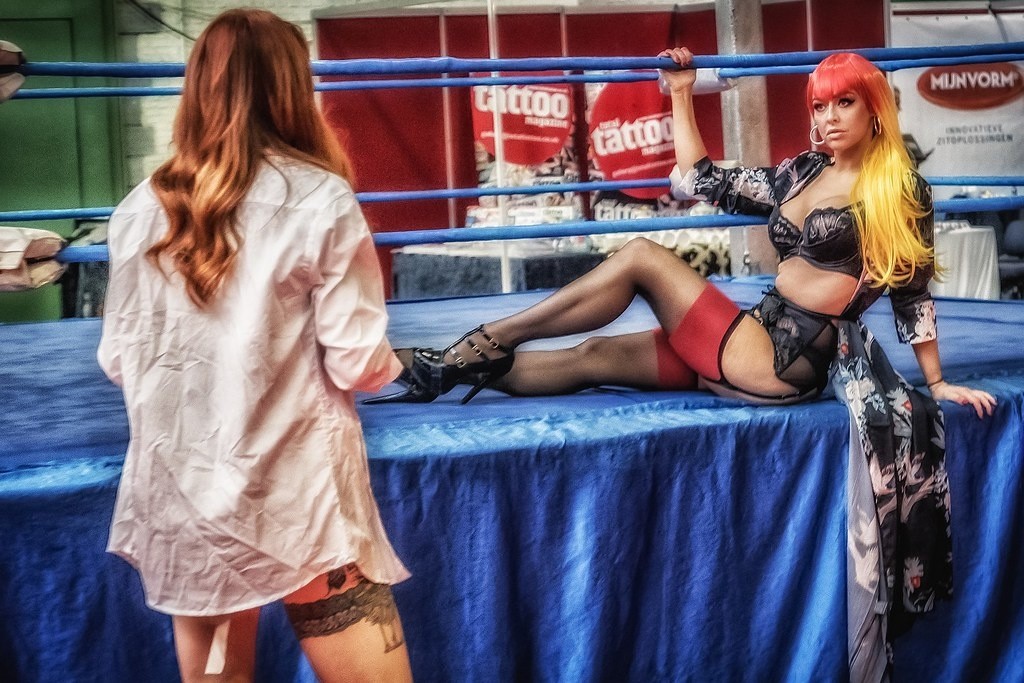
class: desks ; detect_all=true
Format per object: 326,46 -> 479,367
927,220 -> 1001,300
391,242 -> 608,299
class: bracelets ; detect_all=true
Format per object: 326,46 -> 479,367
928,377 -> 945,388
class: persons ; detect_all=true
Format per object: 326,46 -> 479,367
363,43 -> 1001,419
95,8 -> 425,683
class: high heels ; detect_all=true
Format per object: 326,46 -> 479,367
392,323 -> 517,400
363,347 -> 438,406
